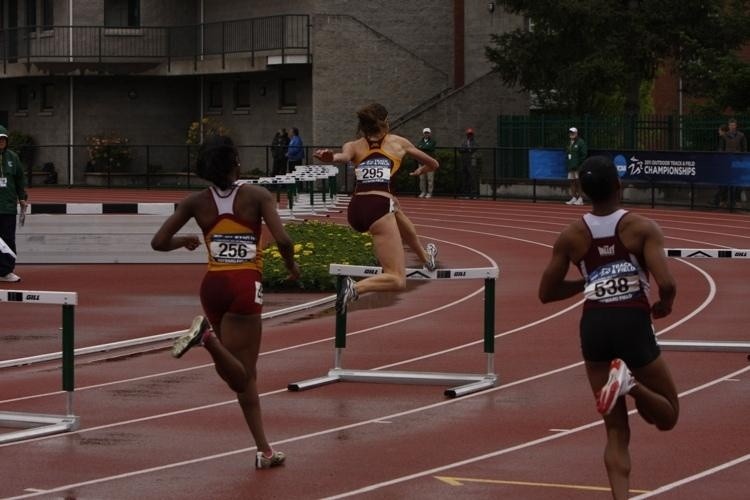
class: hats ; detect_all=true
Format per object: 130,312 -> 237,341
465,128 -> 475,135
569,127 -> 578,133
0,125 -> 8,138
423,128 -> 431,133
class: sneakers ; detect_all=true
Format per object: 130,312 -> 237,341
335,275 -> 358,317
172,315 -> 214,359
596,358 -> 637,416
255,449 -> 285,470
424,193 -> 480,200
573,196 -> 584,205
426,243 -> 438,271
0,273 -> 21,282
419,192 -> 425,197
565,196 -> 577,205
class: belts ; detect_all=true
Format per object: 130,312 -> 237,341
288,159 -> 297,162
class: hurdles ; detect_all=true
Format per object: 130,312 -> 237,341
236,164 -> 352,221
287,265 -> 500,398
0,290 -> 80,444
16,201 -> 209,263
651,247 -> 749,352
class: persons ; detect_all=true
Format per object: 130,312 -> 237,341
284,128 -> 306,176
417,127 -> 437,199
702,119 -> 748,213
270,127 -> 290,176
0,125 -> 29,282
537,153 -> 679,500
458,127 -> 481,200
150,136 -> 302,470
564,127 -> 588,207
312,103 -> 439,314
